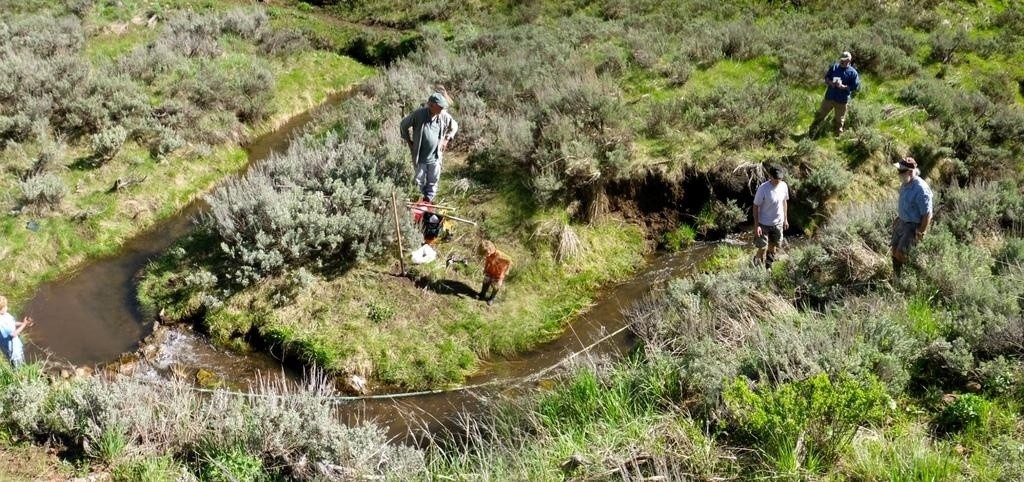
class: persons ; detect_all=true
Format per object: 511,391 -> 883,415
408,196 -> 431,224
475,239 -> 513,306
0,296 -> 34,370
808,50 -> 861,142
749,163 -> 792,273
420,212 -> 455,244
400,92 -> 459,204
888,155 -> 935,283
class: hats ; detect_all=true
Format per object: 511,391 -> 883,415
838,51 -> 852,62
893,157 -> 918,171
428,93 -> 450,109
770,165 -> 785,179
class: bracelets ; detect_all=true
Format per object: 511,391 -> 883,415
753,223 -> 759,227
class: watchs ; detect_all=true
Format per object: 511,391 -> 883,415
444,136 -> 452,142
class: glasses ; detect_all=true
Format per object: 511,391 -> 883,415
897,169 -> 909,174
